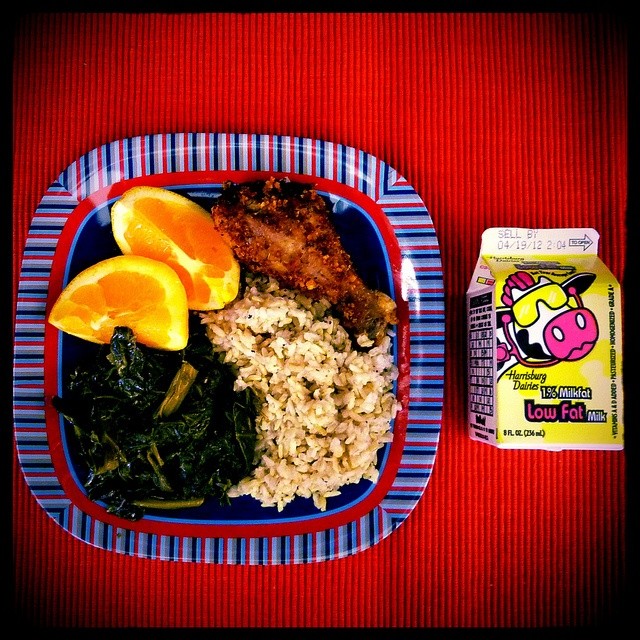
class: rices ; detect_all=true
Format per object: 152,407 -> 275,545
196,274 -> 402,511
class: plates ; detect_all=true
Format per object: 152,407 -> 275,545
13,133 -> 445,566
